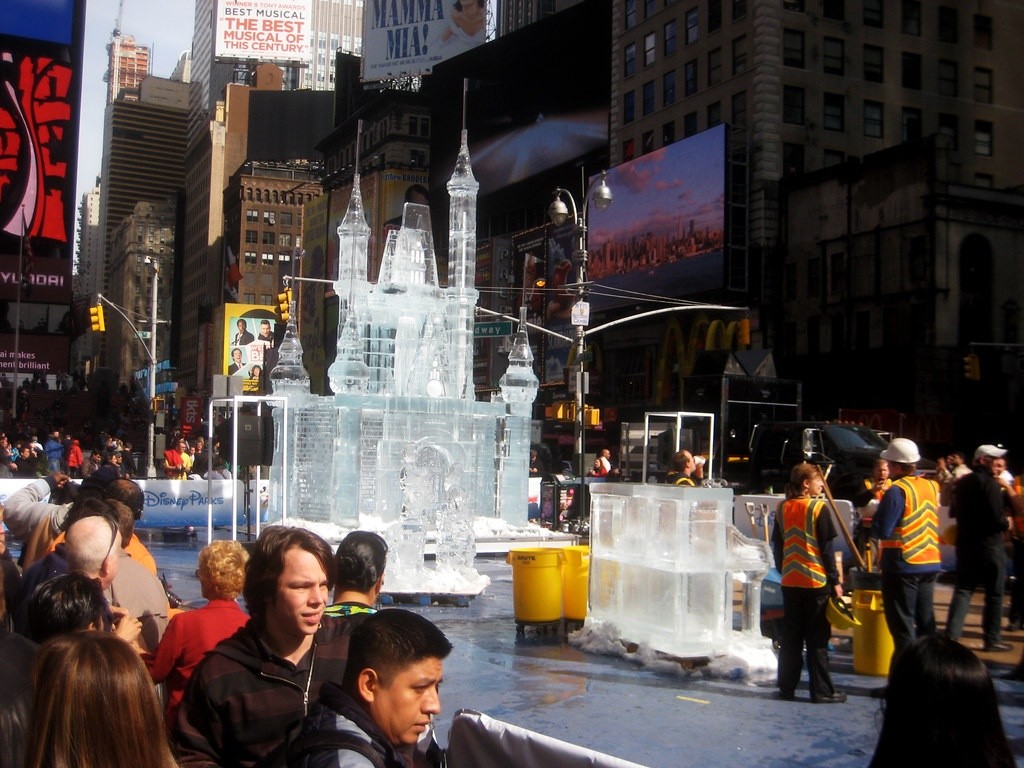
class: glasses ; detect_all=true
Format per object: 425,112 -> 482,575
81,510 -> 118,566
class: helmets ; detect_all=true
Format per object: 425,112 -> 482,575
879,437 -> 921,463
825,596 -> 862,630
857,499 -> 880,517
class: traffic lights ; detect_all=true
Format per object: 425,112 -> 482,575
89,305 -> 105,333
277,290 -> 293,322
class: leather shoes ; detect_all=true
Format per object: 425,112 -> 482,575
985,640 -> 1014,651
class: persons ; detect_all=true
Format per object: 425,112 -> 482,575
228,349 -> 245,376
854,457 -> 896,557
382,182 -> 427,227
530,449 -> 547,478
232,321 -> 254,345
440,0 -> 486,45
588,448 -> 621,482
138,539 -> 253,739
250,366 -> 262,379
773,464 -> 846,703
866,635 -> 1017,768
946,445 -> 1014,651
0,367 -> 235,481
257,319 -> 276,349
170,526 -> 378,768
871,438 -> 941,651
935,454 -> 1016,516
669,449 -> 704,487
322,527 -> 388,617
281,608 -> 453,768
0,458 -> 171,768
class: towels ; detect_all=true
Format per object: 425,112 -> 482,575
164,448 -> 184,479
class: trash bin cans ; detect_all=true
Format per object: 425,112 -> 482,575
850,565 -> 895,675
507,547 -> 567,636
562,546 -> 614,629
540,473 -> 581,525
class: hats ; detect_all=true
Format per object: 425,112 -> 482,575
80,467 -> 120,491
973,445 -> 1008,461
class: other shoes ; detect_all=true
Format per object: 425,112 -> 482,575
779,687 -> 795,699
811,690 -> 847,702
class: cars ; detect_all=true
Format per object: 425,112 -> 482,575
745,417 -> 940,511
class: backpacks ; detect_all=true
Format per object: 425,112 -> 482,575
252,716 -> 386,768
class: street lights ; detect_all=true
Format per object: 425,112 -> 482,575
547,164 -> 613,520
143,254 -> 159,479
